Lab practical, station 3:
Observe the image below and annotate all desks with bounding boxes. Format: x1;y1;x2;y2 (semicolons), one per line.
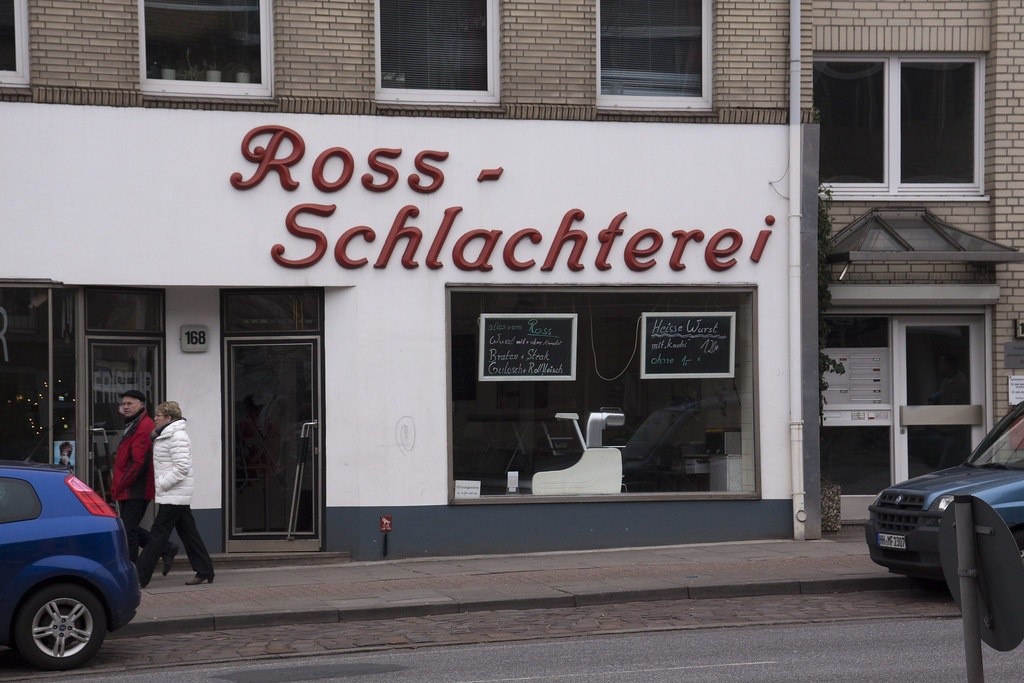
466;405;548;477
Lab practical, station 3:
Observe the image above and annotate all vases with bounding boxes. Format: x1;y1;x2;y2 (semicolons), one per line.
160;68;175;79
206;70;221;81
236;71;250;82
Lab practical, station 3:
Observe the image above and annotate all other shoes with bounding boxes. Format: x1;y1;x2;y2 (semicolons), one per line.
161;544;179;576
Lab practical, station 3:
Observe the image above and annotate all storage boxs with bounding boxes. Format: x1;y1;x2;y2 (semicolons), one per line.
704;427;741;454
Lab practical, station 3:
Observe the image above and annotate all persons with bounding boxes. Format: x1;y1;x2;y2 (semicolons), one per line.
58;442;74;470
138;400;217;589
108;388;179;576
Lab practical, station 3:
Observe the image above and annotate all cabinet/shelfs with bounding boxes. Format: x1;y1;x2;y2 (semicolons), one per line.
686;453;744;493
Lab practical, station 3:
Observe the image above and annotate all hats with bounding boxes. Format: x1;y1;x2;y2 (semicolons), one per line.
119;390;145;403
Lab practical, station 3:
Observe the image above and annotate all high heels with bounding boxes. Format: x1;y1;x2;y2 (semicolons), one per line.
185;572;214;585
139;578;149;588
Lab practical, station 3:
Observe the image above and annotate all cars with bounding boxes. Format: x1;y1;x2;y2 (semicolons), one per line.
865;400;1023;578
0;459;142;672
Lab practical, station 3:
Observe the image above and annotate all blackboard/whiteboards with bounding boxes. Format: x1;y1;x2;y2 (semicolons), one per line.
477;314;578;381
641;312;737;381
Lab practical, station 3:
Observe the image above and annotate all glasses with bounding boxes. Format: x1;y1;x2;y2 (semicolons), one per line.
156;415;165;417
